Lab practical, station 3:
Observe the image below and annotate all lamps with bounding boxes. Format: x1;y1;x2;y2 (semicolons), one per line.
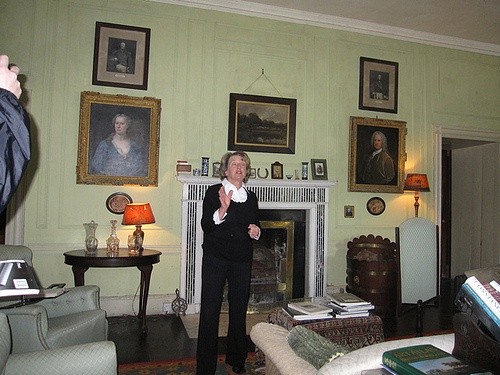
120;203;155;252
403;172;431;217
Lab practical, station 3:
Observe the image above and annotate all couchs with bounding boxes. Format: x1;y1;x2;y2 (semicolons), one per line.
249;321;455;375
0;241;118;375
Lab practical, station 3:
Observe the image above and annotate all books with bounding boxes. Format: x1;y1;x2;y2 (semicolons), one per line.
282;293;375;320
381;344;492;375
454;274;500;343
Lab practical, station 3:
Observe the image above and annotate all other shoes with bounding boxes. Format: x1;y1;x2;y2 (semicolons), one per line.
232;364;246;374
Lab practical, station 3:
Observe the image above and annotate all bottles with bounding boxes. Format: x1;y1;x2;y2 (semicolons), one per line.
83;220;98;255
107;220;120;254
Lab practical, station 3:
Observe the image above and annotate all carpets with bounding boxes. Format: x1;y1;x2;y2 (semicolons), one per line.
180;310;269;339
119;348;256;375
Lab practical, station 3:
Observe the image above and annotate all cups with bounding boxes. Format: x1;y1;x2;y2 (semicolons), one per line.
127;235;142;255
193;169;200;176
286;175;293;179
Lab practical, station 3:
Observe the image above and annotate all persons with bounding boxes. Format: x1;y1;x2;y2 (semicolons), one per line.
90;113;146;178
111;42;133;73
197;152;262;375
0;55;31;215
361;131;394;184
375;74;383;100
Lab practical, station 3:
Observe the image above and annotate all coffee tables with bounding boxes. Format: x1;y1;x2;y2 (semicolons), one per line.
265;305;385;352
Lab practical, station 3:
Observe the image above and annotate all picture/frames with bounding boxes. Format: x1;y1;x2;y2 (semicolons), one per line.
366;196;386;216
106;192;133;215
88;21;152;90
357;56;399;114
343;205;355;219
227;93;298;154
311;158;328;181
271;162;283;180
347;116;408;195
75;91;162;188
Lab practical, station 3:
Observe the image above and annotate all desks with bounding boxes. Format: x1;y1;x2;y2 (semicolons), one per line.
63;248;162;335
